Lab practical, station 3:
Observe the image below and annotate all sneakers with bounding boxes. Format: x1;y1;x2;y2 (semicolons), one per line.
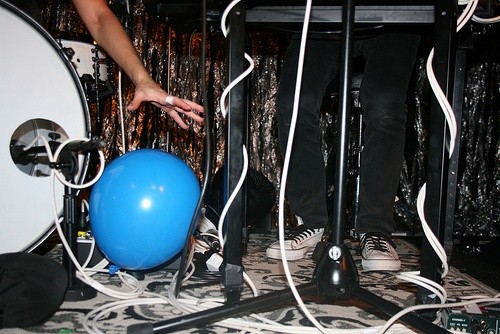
358;231;401;272
267;227;325;260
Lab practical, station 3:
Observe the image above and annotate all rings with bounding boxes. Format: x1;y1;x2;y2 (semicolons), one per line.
166;95;174;105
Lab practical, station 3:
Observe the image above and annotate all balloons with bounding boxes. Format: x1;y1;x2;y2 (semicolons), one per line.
89;149;202;276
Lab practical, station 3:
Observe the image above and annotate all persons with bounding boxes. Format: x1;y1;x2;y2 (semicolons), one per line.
73;0;204;130
265;0;425;271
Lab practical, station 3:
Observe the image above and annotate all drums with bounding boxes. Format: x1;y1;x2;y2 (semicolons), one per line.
54;32;116;102
0;0;92;255
